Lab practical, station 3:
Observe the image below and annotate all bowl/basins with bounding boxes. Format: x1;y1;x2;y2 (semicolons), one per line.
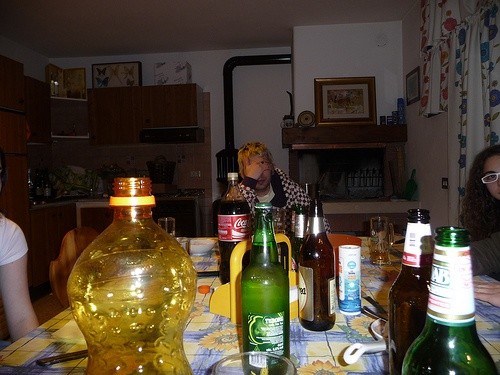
327;233;362;276
189;240;215;256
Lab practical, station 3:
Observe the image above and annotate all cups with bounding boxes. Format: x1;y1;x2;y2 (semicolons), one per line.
157;216;176;238
369;217;394;265
211;350;297;375
380;98;405;125
280;118;293;128
272;208;286;234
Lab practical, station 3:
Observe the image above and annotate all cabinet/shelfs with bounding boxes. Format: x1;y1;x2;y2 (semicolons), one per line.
0;54;212;295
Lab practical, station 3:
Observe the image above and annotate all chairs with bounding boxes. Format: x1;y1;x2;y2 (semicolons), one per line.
50;227;97;310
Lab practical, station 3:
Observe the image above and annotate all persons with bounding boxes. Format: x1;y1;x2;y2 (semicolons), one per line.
0;214;40;350
458;144;500;307
227;142;331;234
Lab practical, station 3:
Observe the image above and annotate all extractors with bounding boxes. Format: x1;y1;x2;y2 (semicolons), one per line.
138;127;205;144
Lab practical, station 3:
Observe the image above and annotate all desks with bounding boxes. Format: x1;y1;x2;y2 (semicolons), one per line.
0;233;500;374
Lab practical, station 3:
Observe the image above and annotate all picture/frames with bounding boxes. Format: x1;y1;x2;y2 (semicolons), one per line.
313;77;377;125
91;61;142;87
406;65;420;105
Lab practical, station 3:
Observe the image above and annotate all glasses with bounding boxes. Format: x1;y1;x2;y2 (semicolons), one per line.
481;173;500;184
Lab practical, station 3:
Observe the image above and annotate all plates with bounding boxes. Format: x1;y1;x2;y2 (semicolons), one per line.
298;111;315;127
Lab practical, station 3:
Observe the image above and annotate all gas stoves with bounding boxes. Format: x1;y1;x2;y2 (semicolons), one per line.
152;188;204;201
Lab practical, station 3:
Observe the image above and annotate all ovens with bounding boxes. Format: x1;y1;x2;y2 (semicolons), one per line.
152;201;200;239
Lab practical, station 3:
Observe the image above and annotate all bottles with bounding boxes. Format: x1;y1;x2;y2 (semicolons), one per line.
298;199;337;332
65;176;197;375
27;168;53;206
217;172;251;285
303;181;312;197
292;206;307;272
242;202;290;375
388;208;433;375
71;120;78;136
291;203;301;247
402;226;499;375
